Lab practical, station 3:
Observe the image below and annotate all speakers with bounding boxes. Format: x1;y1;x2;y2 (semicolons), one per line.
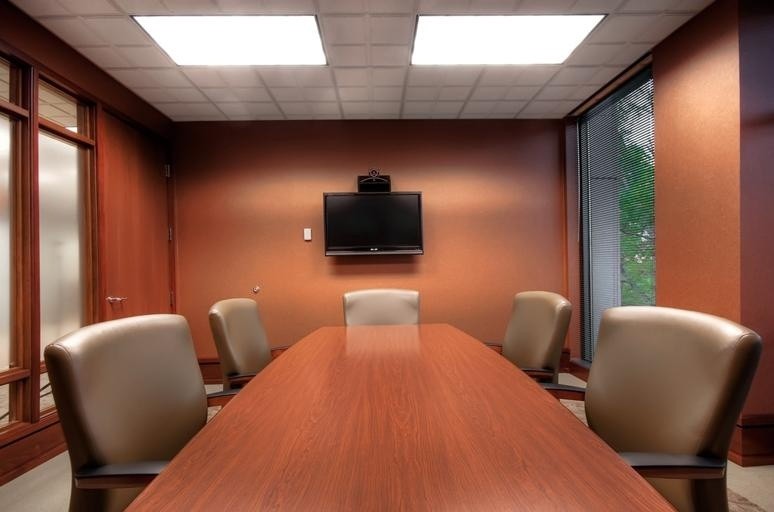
358;175;391;192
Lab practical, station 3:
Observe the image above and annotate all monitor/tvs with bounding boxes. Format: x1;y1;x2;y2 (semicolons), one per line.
323;192;424;256
